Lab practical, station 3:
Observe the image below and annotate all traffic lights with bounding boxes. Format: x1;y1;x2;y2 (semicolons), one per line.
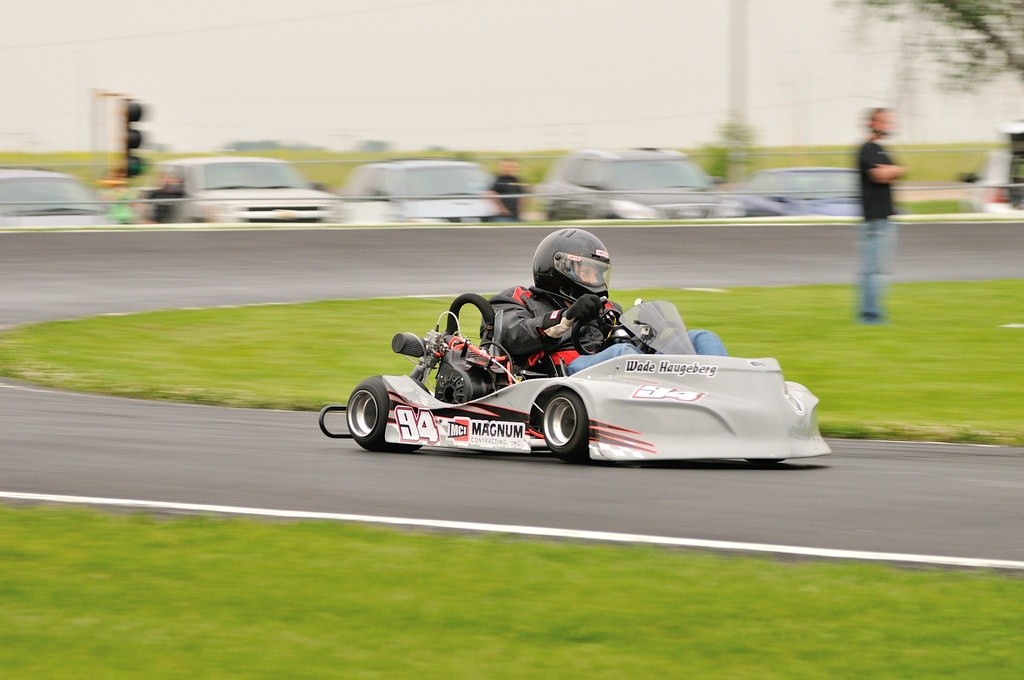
108;96;144;178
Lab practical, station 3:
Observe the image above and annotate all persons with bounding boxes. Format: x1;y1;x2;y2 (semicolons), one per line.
858;106;908;326
487;160;532;222
480;227;728;377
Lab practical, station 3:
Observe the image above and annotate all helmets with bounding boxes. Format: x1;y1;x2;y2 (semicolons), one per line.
533;229;610;305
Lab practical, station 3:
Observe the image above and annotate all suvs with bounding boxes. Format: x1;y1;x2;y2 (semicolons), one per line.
539;138;751;218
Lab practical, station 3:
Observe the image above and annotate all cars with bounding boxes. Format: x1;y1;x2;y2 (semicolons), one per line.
744;162;899;216
148;157;348;227
1;165;117;226
979;119;1024;214
341;160;511;226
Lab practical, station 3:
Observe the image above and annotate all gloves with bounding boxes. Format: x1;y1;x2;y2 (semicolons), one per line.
566;294;602;326
637;303;665;338
